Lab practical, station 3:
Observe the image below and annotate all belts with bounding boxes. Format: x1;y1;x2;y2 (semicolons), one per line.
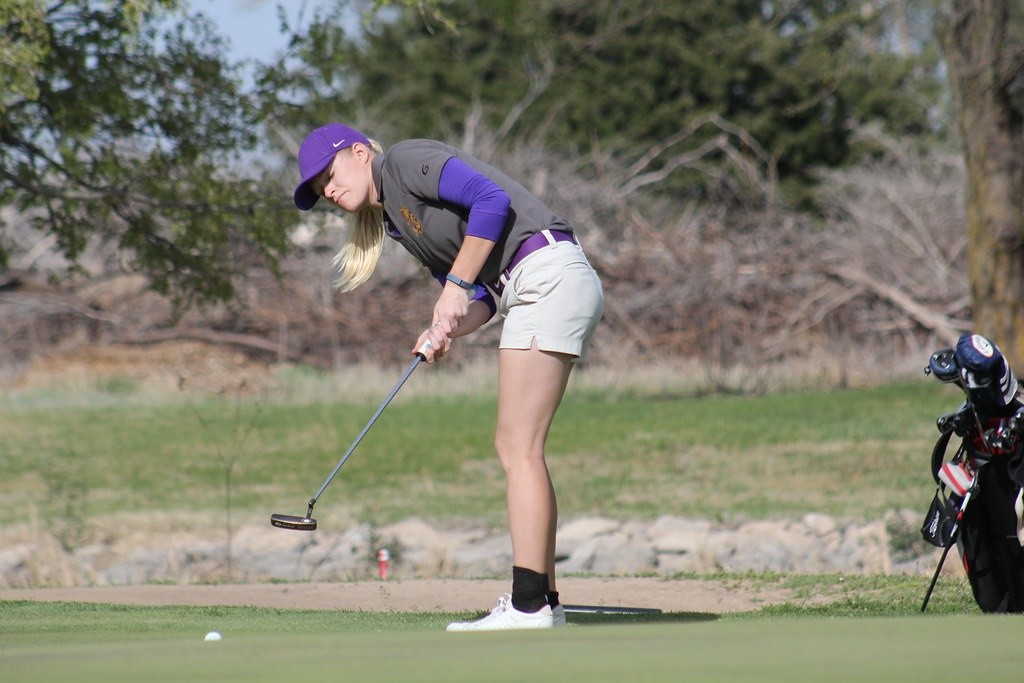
489;228;578;298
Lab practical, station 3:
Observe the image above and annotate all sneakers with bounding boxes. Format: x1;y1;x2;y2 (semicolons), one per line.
550;603;566;626
445;591;554;632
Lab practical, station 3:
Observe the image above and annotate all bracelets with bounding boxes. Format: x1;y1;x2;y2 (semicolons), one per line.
446;274;472;290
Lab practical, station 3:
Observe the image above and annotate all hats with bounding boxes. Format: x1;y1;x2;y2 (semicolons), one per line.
293;123;369;211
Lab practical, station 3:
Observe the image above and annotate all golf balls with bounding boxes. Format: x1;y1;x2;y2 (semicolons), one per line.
204;632;221;641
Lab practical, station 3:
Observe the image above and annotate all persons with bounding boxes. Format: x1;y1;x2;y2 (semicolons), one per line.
293;123;603;631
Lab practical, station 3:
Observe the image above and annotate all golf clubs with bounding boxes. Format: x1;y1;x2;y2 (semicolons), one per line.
270;338;433;532
928;331;1024;458
